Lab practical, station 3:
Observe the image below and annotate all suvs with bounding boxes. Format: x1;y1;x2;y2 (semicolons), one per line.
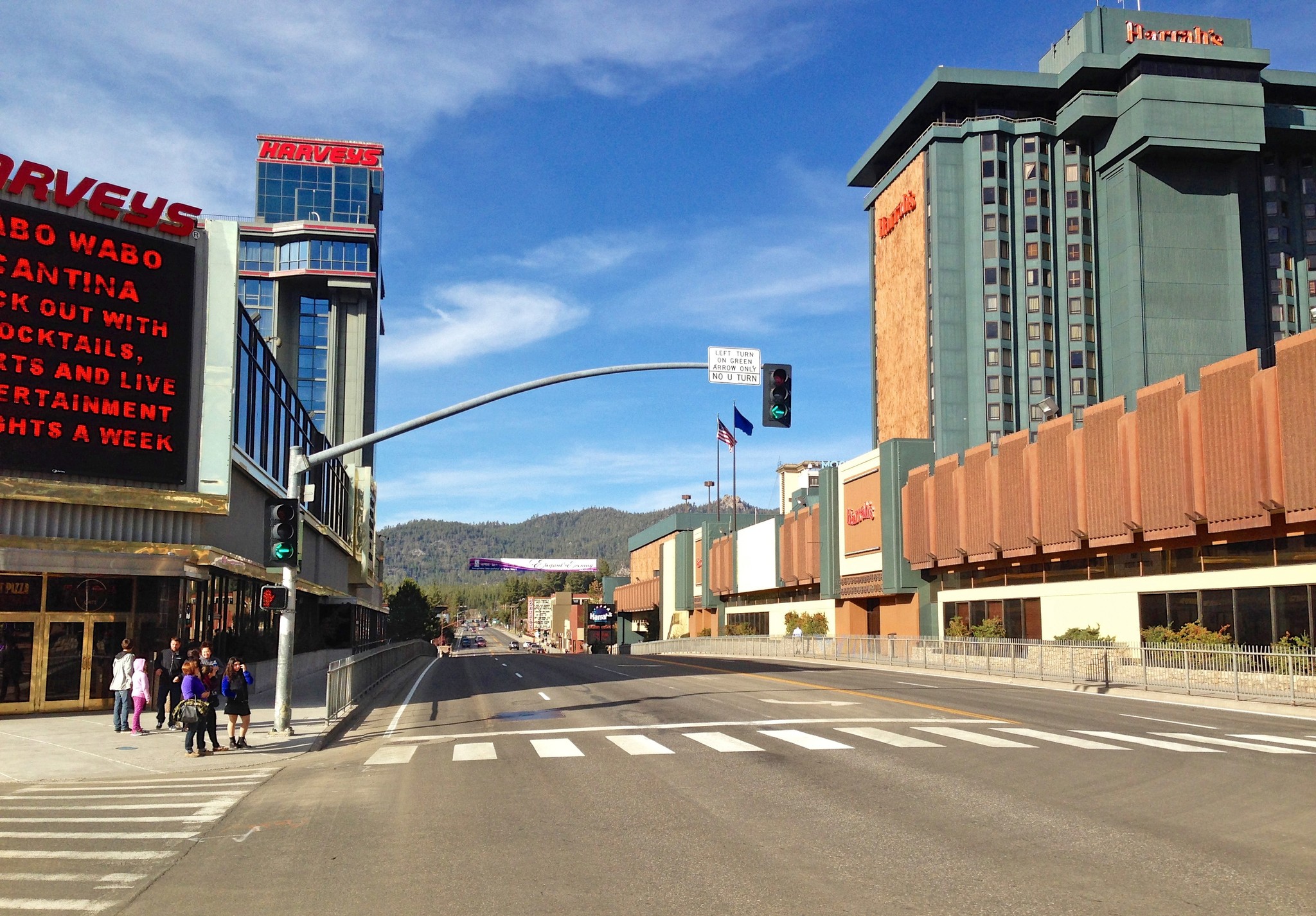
509;641;519;650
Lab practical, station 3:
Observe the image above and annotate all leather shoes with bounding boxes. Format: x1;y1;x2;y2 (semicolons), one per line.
198;750;214;756
185;751;200;758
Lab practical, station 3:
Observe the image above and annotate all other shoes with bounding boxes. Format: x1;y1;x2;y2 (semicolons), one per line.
212;746;228;752
182;727;188;732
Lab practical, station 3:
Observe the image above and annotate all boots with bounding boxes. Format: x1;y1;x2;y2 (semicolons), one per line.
230;736;240;749
237;736;252;748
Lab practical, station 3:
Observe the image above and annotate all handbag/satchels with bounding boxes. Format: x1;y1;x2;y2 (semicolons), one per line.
177;693;199;724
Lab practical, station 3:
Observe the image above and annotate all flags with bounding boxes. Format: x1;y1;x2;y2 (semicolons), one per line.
734;406;753;436
717;418;738;453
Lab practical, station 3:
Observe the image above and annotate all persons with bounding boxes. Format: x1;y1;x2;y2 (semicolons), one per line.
621;642;625;645
448;646;451;652
221;656;252;749
198;640;224;679
792;625;804;654
547;641;557;648
182;648;202;679
153;636;186;731
181;660;214;758
130;657;151;737
638;640;643;643
541;638;544;643
517;631;522;636
198;665;228;752
109;637;136;734
0;625;24;703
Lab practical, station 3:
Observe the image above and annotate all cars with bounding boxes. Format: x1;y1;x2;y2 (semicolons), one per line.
475;636;487;648
461;636;470;648
523;641;544;654
464;625;486;633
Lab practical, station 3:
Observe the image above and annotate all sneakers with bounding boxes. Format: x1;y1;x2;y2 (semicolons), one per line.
139;729;150;734
115;729;121;733
169;725;177;731
131;731;143;736
156;722;163;730
120;728;133;733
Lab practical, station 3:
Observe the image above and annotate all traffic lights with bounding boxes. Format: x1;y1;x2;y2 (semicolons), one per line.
263;497;298;568
260;585;289;610
762;362;793;427
